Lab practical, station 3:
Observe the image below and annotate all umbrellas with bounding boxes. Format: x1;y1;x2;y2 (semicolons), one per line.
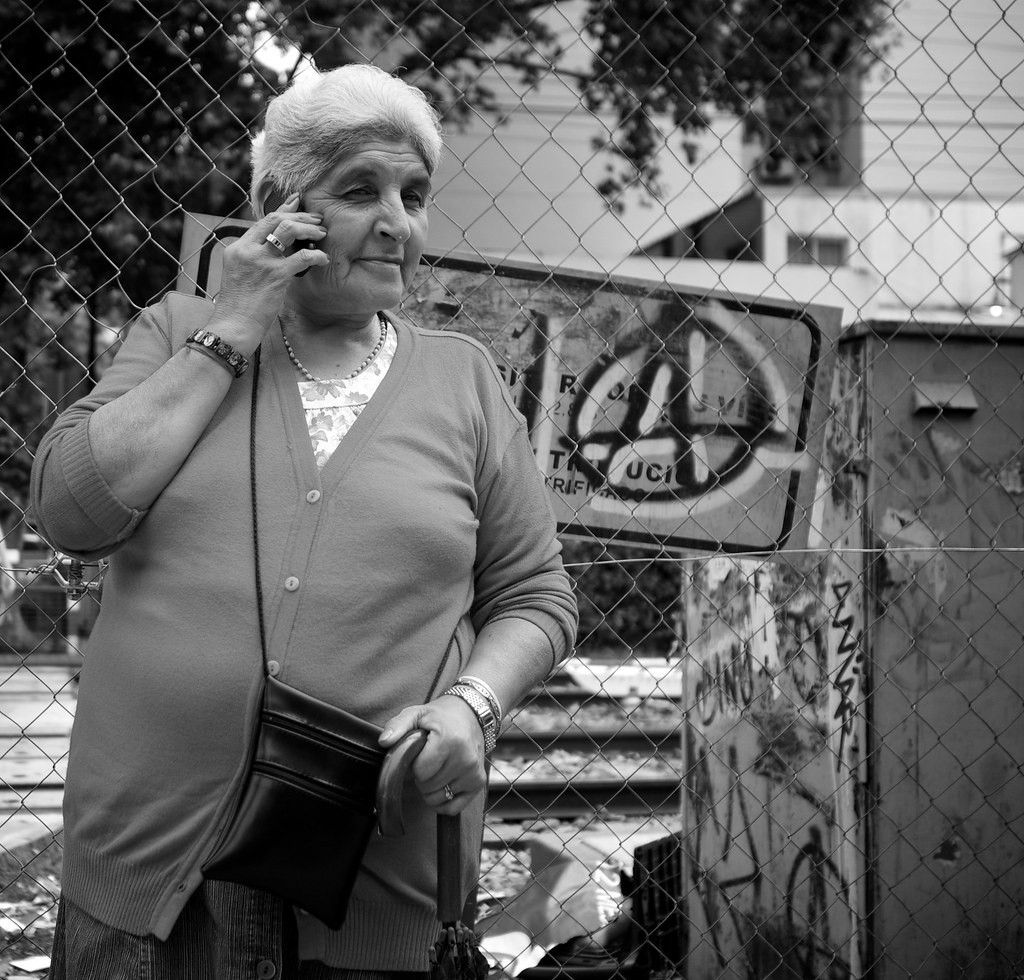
376;728;490;980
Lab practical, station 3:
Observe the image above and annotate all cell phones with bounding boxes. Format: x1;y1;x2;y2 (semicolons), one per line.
264;188;317;278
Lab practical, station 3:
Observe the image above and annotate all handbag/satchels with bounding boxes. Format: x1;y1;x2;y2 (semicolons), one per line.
199;675;398;933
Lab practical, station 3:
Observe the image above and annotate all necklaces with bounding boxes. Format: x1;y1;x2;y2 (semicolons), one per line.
283;317;385;381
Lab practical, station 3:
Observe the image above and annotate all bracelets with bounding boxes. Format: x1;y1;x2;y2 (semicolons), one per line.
182;327;250;381
444;675;502;756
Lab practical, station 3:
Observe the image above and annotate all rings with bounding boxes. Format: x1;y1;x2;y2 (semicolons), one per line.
266;233;286;253
444;784;456;800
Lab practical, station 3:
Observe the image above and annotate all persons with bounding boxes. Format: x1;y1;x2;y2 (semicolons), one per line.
29;63;579;980
